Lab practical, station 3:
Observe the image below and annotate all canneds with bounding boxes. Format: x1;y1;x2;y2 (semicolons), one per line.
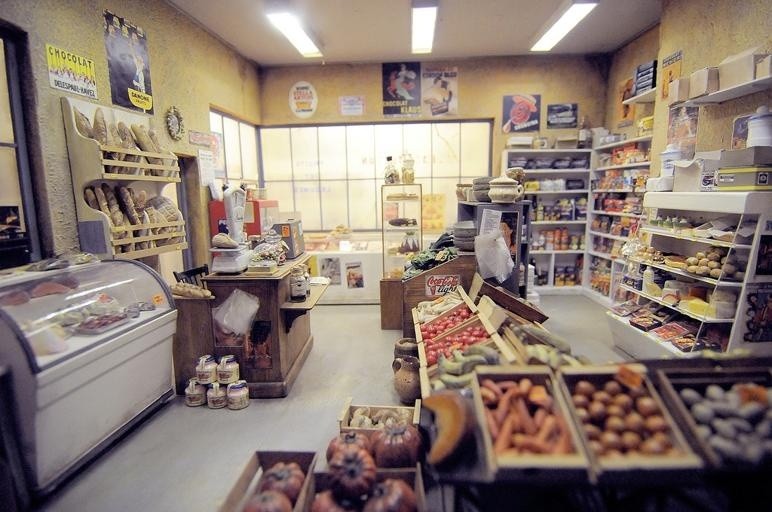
184;355;250;410
540;230;554;250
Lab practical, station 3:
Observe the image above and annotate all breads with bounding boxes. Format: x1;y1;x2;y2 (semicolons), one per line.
69;107;185;255
211;233;239;248
687;246;738;279
170;283;212;299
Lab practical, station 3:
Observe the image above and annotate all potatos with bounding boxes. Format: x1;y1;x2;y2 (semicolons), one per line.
572;381;672;460
681;383;772;462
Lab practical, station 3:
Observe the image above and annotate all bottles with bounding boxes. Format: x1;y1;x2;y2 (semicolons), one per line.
530;256;547;284
529;197;544;221
577;115;591;146
456;184;473;201
542;227;584;250
642;264;654;291
385;153;414;182
291;264;310;304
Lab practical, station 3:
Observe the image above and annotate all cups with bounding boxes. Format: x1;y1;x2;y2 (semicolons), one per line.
488;176;524;204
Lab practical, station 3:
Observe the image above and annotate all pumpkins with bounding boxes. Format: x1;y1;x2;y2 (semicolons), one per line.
244;422;423;512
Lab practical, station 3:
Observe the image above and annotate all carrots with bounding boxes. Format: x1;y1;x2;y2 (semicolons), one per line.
479;378;572;454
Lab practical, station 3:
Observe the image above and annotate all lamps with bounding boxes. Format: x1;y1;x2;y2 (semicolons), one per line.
266;5;326;59
412;3;438;54
528;1;598;52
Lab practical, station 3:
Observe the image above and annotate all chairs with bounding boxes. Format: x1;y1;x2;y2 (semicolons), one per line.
172;264;210;296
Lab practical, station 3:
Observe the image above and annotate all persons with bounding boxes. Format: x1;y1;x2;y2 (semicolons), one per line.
424;77;451;105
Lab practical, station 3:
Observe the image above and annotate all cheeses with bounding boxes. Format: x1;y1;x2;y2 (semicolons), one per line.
644;277;736;318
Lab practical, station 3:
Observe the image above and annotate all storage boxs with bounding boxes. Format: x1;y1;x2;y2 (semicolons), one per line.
667;49;771;106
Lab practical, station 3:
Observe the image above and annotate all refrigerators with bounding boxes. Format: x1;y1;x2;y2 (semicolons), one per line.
3;257;177;489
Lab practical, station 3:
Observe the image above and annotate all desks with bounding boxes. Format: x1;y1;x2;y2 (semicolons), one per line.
201;253;314;398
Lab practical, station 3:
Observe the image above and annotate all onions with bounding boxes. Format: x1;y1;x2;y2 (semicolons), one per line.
419;306;489;366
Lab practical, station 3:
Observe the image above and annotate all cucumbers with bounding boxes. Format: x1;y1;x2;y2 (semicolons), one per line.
430;345;499;392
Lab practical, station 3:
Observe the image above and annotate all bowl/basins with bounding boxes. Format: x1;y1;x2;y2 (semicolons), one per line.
512;156;587;169
472;178;492;202
452;222;477;250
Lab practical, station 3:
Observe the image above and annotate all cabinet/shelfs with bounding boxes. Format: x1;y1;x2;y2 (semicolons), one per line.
607;188;770;364
582;131;658;311
60;96;194;259
501;150;589;297
383;183;423;283
